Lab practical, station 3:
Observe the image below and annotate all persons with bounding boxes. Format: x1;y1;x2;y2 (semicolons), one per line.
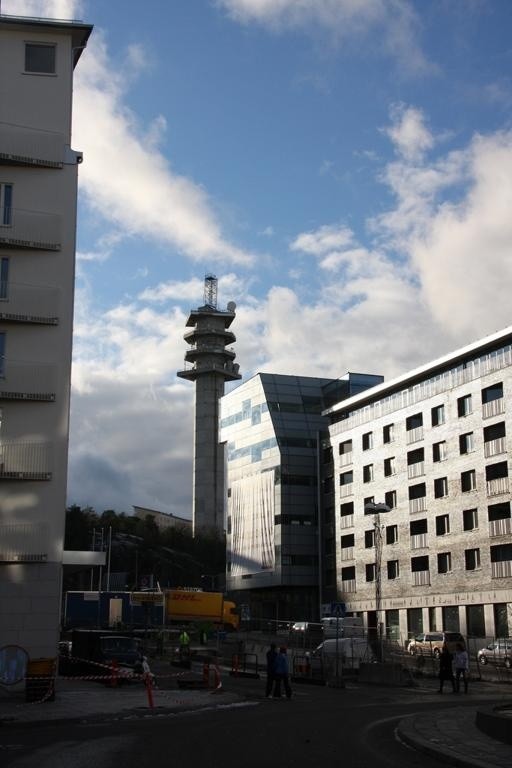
435;646;458;693
450;642;470;694
261;643;283;700
273;647;295;699
179;628;191;656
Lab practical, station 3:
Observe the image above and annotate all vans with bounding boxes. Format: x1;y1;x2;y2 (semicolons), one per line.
291;615;375;660
405;631;467;660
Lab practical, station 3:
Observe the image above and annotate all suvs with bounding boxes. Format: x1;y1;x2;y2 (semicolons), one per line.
476;636;511;667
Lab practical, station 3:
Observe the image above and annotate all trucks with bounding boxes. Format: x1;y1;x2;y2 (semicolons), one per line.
166;589;239;634
96;634;145;682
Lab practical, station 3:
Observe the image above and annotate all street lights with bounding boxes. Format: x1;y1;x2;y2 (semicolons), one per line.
364;501;391;647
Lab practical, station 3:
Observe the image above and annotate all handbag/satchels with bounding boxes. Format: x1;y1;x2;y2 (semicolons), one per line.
464;672;470;678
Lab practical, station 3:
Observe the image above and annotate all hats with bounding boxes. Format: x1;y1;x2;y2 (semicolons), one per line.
280;646;287;652
